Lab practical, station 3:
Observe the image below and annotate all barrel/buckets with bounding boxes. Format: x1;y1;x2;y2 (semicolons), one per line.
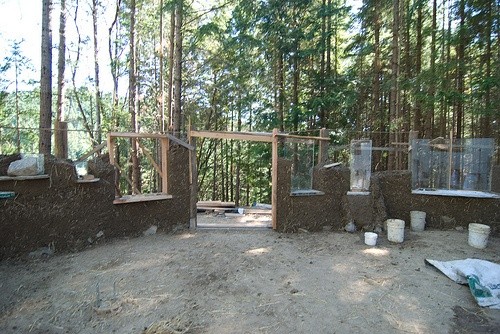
468;223;490;249
387;219;406;243
410;211;427;232
364;232;378;246
